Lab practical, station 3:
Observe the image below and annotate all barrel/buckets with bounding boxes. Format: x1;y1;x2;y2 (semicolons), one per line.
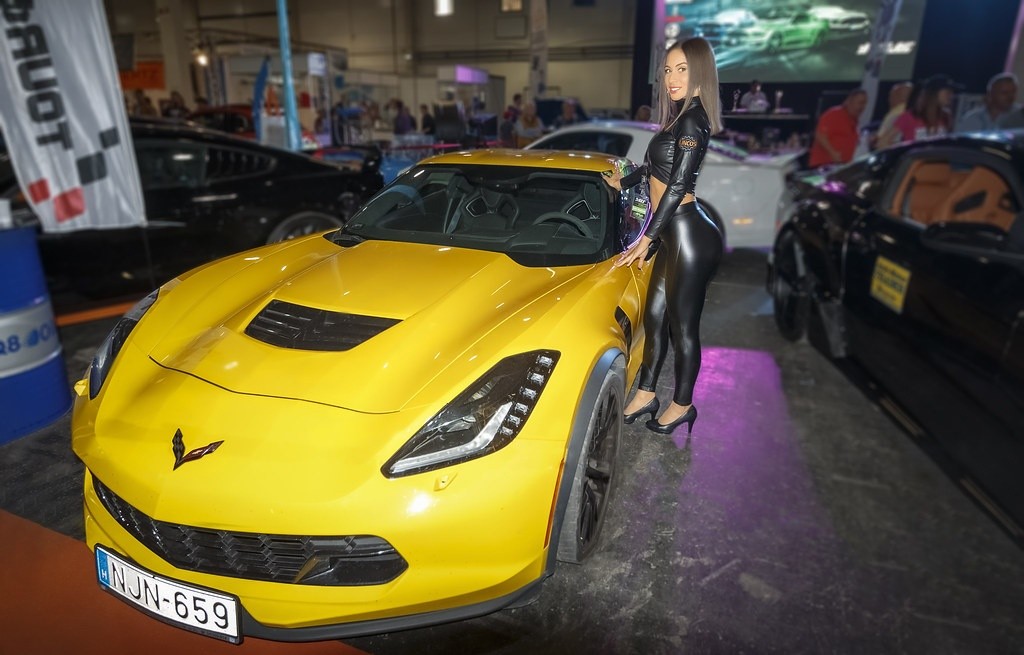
0;222;73;444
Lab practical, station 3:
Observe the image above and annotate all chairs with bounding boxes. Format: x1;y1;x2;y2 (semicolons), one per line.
560;182;600;238
904;165;1008;228
453;187;520;235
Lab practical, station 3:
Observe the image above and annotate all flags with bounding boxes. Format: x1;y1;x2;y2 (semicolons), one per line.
0;0;148;233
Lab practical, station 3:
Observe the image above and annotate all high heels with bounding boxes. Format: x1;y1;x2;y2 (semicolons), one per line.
621;396;660;424
646;405;697;434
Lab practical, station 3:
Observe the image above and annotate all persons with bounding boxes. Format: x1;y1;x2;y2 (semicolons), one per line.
124;86;582;150
742;72;1024;168
602;37;724;435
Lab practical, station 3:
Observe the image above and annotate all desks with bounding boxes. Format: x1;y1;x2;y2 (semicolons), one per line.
385;144;461;155
477;141;509;148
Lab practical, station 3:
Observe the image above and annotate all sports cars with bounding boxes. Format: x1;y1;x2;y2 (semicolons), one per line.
72;146;661;646
523;120;808;254
767;129;1024;553
0;117;382;315
